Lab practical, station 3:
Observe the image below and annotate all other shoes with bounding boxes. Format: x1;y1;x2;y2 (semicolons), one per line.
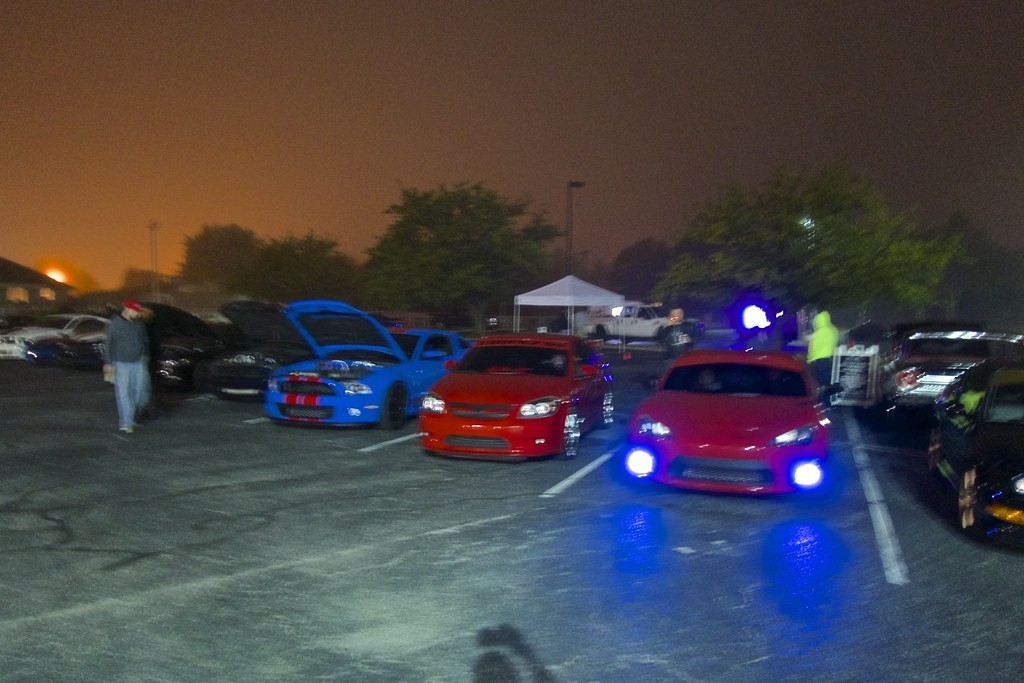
822;406;831;413
120;427;134;434
133;422;145;429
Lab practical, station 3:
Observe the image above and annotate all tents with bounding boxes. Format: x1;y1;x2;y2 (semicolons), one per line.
513;274;626;356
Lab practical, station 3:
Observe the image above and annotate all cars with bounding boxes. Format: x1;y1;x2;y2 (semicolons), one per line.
417;332;613;459
215;298;316;404
0;313;111;361
630;347;843;495
24;304;208;372
150;332;233;387
265;299;478;429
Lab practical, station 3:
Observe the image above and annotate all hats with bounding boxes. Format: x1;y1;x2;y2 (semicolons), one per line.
125;301;144;312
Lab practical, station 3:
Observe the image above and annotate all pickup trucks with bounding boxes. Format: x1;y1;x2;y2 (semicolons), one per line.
574;304;702;347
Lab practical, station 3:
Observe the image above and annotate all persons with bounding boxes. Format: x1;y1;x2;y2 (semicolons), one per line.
806;310;839;413
657;305;697;363
105;301;150;433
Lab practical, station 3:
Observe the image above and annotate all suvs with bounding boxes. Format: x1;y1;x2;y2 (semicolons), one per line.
877;322;991;430
928;359;1024;540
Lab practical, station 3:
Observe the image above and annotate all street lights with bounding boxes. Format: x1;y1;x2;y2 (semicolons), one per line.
564;181;586;274
